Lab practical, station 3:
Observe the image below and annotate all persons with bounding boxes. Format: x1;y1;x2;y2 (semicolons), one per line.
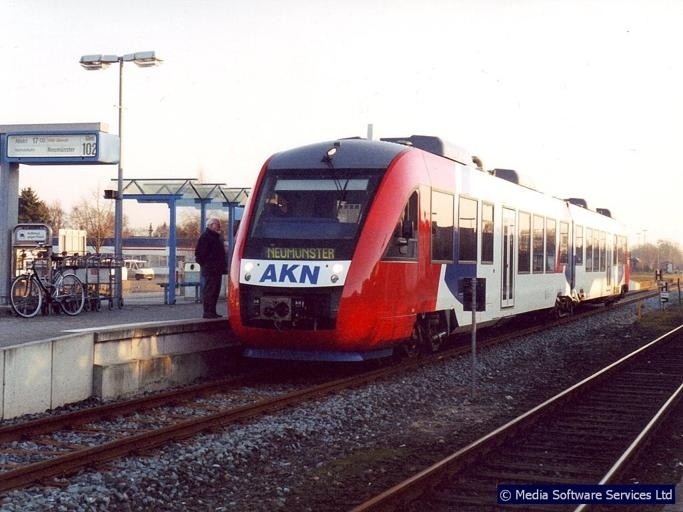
194;218;225;319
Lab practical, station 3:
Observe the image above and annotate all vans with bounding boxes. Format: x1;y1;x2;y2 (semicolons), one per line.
122;260;155;281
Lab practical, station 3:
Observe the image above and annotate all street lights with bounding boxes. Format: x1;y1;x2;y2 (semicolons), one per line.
78;50;165;305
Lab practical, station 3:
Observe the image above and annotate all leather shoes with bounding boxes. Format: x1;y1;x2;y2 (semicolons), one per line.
203;313;223;319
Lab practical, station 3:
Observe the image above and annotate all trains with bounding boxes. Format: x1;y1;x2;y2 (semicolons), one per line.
226;135;631;382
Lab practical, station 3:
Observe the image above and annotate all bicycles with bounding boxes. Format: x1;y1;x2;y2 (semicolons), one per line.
10;258;85;318
